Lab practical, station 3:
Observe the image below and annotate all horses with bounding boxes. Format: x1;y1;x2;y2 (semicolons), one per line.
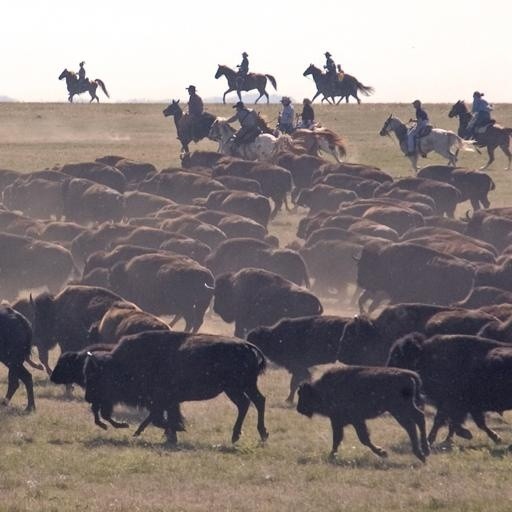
302;64;373;104
447;99;512;170
58;68;109;104
378;113;477;172
215;64;277;105
206;111;346;159
162;98;215;153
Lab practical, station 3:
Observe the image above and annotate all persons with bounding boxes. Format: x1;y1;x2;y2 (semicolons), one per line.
235;52;249;89
405;100;430;156
338;64;344;74
224;101;256;153
76;62;85;86
324;52;337;78
297;99;315;128
277;97;294;133
464;92;492;144
184;86;203;121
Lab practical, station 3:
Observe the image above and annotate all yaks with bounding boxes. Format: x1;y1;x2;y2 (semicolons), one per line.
0;150;512;466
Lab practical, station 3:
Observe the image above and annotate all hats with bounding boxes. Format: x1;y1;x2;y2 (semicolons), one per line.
232;101;246;108
303;98;312;105
323;51;332;56
279;96;292;103
413;99;420;106
186;85;198;91
242;51;249;57
